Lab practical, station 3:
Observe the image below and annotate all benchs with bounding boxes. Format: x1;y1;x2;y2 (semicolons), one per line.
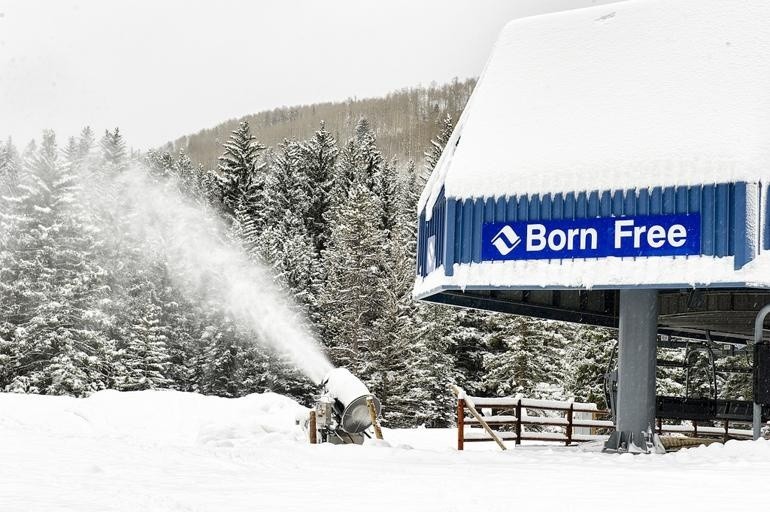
655;395;717;437
715;396;770;437
